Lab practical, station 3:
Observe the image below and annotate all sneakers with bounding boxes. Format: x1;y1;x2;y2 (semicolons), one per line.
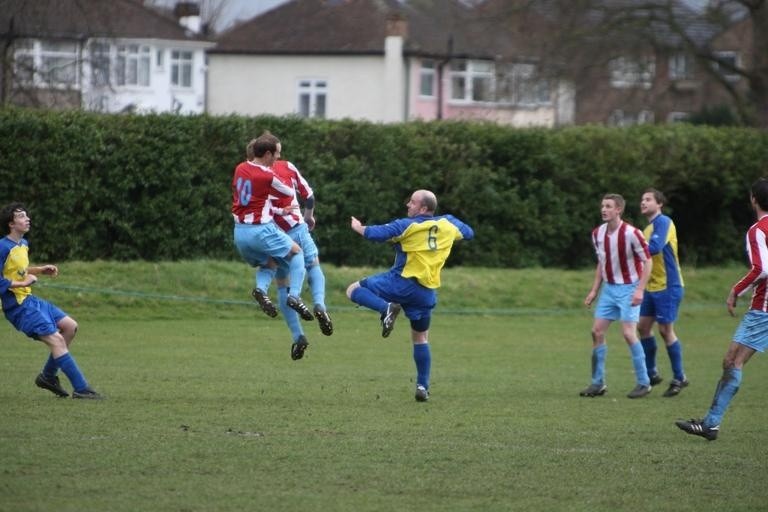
33;370;102;401
413;385;431;403
626;374;690;399
578;383;609;398
379;299;401;339
251;287;334;361
673;416;720;441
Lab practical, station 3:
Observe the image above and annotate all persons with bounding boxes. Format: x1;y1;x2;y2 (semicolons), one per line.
636;188;689;397
675;178;768;442
0;203;102;400
345;188;475;402
232;133;315;322
579;191;653;398
245;138;334;361
